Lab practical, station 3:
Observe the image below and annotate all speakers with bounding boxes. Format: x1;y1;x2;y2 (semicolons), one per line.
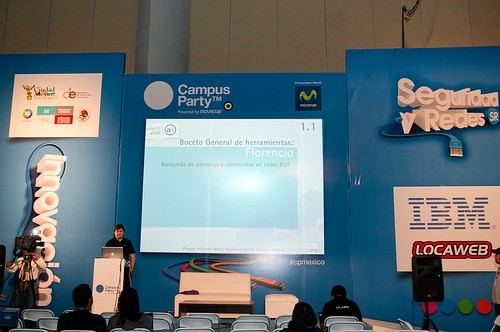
412;255;444;302
0;245;6;292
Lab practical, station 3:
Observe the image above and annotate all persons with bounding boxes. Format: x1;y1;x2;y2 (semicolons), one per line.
57;283;107;332
490;247;500;332
6;251;46;311
320;285;363;329
279;302;323;332
107;287;154;330
105;223;137;288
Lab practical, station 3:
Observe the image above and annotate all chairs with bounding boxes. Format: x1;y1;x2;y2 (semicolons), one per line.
491;315;500;332
9;309;430;332
266;294;298;318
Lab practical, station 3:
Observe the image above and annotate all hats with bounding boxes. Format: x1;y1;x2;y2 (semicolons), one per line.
491;247;500;254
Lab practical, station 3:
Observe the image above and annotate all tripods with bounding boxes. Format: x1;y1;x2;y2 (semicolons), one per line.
8;256;36;308
421;302;439;332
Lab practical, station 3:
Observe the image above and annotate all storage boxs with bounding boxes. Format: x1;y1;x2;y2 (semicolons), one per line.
2;308;20;326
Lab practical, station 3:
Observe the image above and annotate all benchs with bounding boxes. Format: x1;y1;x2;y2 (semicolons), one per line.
174;272;254;317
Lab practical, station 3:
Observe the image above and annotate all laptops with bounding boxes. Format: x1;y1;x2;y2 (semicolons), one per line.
102;247;123;259
145;312;153;331
